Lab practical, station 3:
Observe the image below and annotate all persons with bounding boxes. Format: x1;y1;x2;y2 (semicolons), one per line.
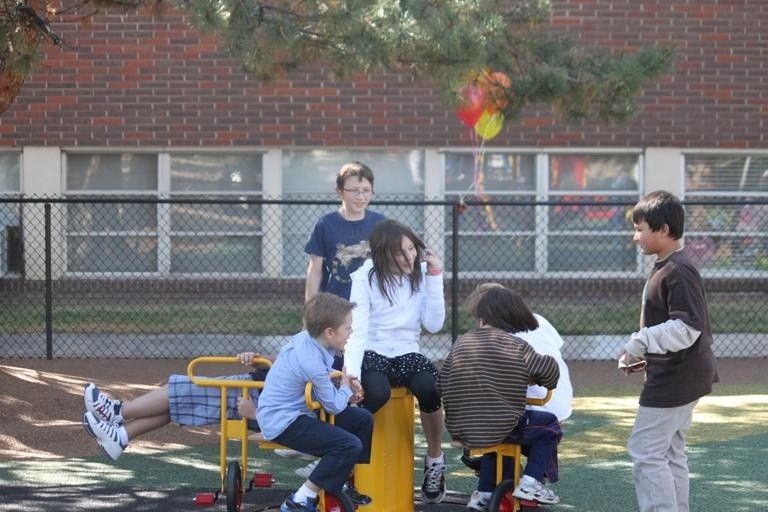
617;190;720;512
461;312;573;477
341;219;447;505
83;352;343;462
434;282;563;512
304;160;390;305
256;292;374;512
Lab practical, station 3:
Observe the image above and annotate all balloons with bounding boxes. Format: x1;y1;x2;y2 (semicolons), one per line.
453;82;486;129
479;72;513;116
474;109;504;141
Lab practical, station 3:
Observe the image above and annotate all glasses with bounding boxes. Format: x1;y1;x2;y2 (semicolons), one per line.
342;187;372;197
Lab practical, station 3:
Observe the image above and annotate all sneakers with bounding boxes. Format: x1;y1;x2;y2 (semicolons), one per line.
280;492;321;512
82;411;127;461
512;475;560;505
295;461;320;478
341;481;372;506
273;448;318;459
467;490;492;511
422;452;446;505
84;383;123;426
